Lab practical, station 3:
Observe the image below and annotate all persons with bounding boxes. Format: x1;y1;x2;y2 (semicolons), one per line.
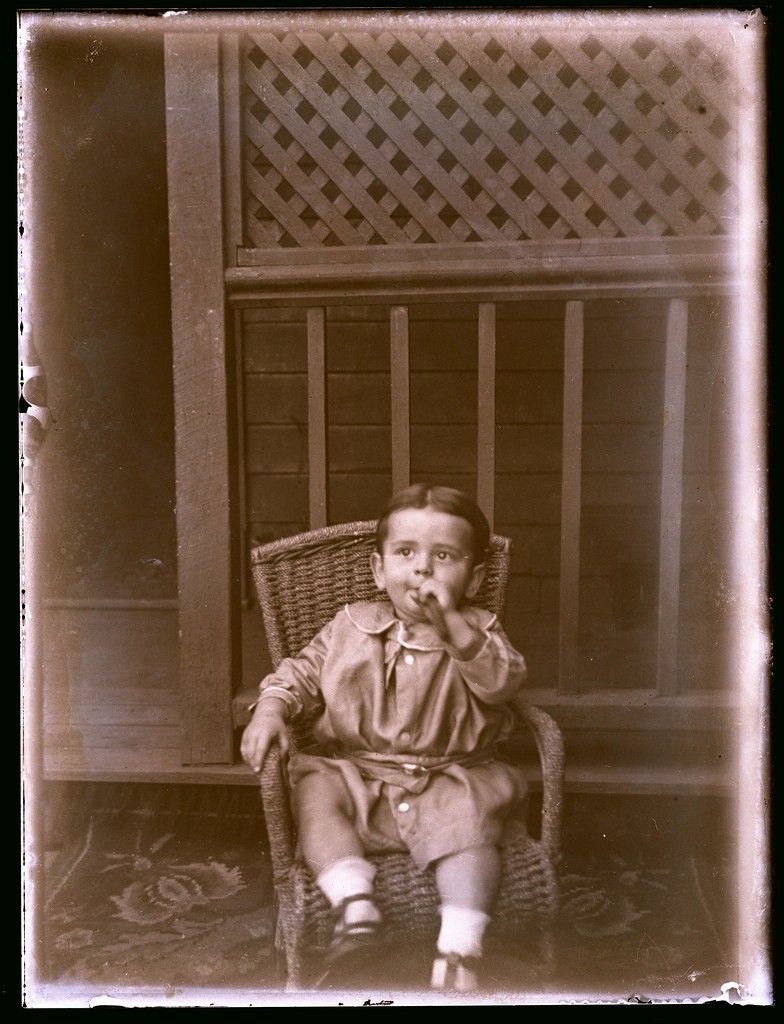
240;482;526;993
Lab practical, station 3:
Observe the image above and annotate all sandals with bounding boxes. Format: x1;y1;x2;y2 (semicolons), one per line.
319;894;392;976
430;945;483;994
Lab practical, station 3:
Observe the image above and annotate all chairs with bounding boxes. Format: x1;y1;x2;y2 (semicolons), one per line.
251;518;564;993
